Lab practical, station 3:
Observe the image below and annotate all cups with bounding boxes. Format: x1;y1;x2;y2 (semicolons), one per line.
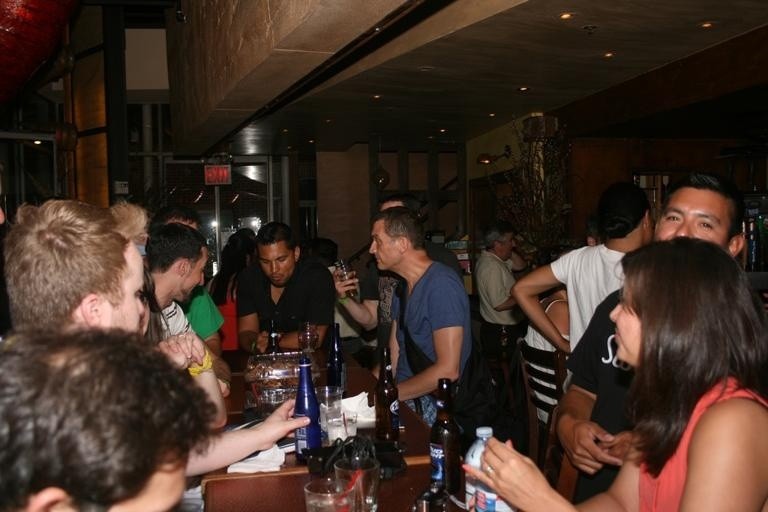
305;457;382;512
332;258;358;297
242;350;360;452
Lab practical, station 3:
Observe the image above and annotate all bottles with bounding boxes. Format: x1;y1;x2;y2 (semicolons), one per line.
324;321;347;397
428;377;460;495
295;356;321;462
295;321;319;356
265;319;283;354
464;424;521;512
414;481;450;511
372;347;403;468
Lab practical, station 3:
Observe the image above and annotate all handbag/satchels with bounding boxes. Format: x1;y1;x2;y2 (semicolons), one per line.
482;338;527;454
397;277;495;418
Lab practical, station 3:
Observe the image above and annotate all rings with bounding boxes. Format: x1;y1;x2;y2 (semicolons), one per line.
485;466;494;475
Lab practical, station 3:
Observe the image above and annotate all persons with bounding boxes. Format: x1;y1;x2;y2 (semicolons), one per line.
584;213;602;247
552;167;768;504
516;245;577;426
475;226;525;420
0;198;312;479
0;327;218;512
140;204;337;397
510;179;655;355
461;234;768;511
332;193;473;417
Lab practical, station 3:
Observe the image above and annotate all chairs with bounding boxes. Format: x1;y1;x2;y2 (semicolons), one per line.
480;325;580;505
